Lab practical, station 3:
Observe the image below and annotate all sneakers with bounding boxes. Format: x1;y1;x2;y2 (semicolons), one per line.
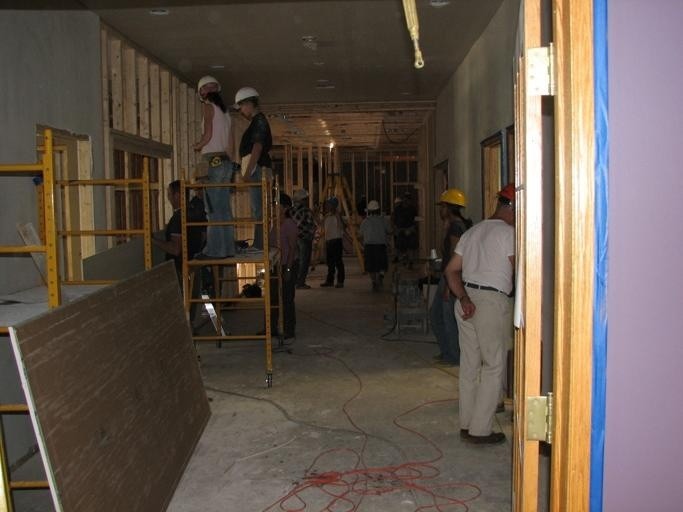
297;284;311;289
460;429;505;444
320;283;343;288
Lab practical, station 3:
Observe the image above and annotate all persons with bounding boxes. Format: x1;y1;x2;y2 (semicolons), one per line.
150;179;315;350
358;195;416;294
231;86;279;256
496;267;516;424
439;182;520;450
427;188;475;366
190;74;238;262
316;198;346;287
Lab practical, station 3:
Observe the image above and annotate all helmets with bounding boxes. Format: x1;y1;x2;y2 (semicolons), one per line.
198;76;259;103
328;197;338;207
498;184;515;202
367;201;379;210
294;189;307;201
440;189;465;207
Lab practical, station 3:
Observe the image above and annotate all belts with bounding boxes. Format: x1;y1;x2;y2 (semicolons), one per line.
463;280;499;291
